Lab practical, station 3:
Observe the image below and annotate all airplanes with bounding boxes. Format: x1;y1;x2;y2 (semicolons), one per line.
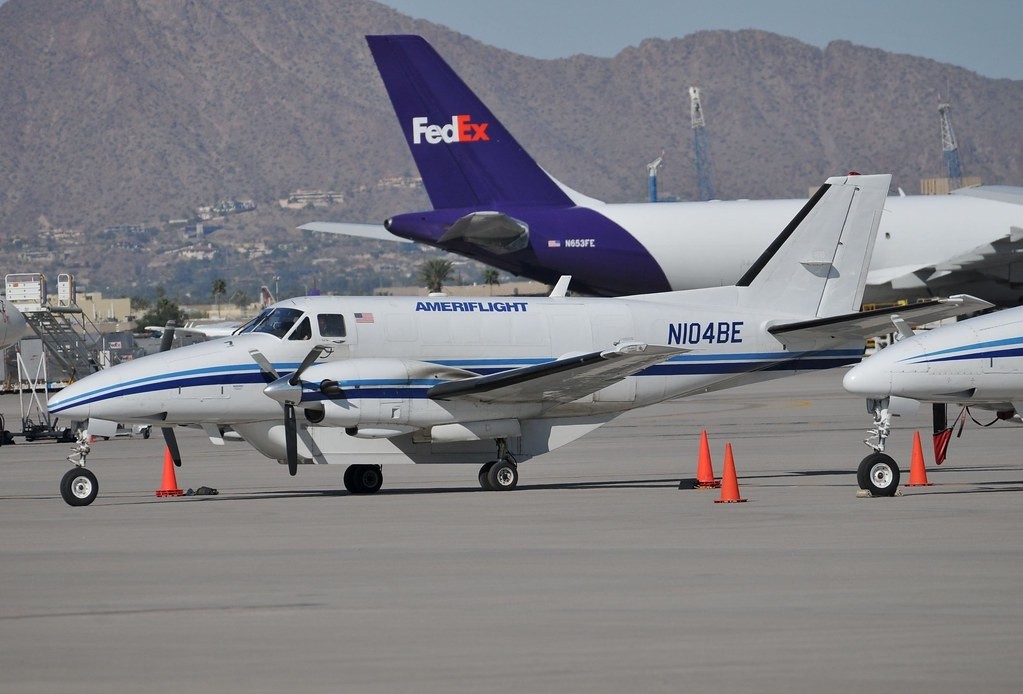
843;304;1023;497
43;172;994;508
299;33;1023;297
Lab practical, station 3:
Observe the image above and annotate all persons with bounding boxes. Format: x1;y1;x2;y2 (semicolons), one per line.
319;318;330;335
289;316;309;340
262;295;270;310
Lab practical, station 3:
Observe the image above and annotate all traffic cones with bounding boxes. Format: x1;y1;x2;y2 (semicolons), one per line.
156;444;183;497
905;430;934;487
714;442;748;503
695;429;722;488
